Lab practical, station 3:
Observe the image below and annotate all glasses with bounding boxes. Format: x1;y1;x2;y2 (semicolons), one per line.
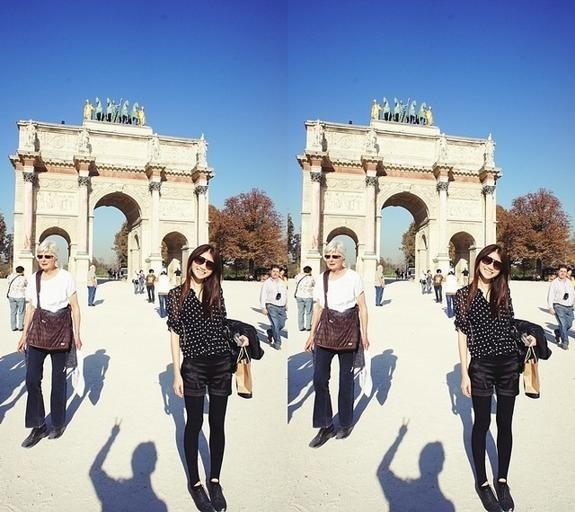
482;255;503;270
324;254;341;259
37;255;53;259
194;255;216;271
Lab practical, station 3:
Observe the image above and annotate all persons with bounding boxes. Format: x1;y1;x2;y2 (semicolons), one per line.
279;268;288;288
262;265;289;350
453;245;537;512
371;97;433;126
566;268;575;287
107;268;124;281
395;268;411;280
84;98;145;126
7;266;27;331
420;268;459;317
17;241;82;448
86;265;97;306
375;263;385;306
547;265;575;350
165;245;250;512
134;269;169;317
293;267;315;331
303;241;369;448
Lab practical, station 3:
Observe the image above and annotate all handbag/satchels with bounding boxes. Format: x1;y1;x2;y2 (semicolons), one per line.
26;306;72;351
523;345;540;395
314;303;359;351
235;347;253;394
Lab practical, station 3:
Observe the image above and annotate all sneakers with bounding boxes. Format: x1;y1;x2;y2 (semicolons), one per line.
190;483;214;511
21;423;49;447
206;481;226;512
267;329;273;342
335;423;353;438
48;424;65;439
477;482;502;511
309;424;335;448
554;329;561;341
495;478;514;511
